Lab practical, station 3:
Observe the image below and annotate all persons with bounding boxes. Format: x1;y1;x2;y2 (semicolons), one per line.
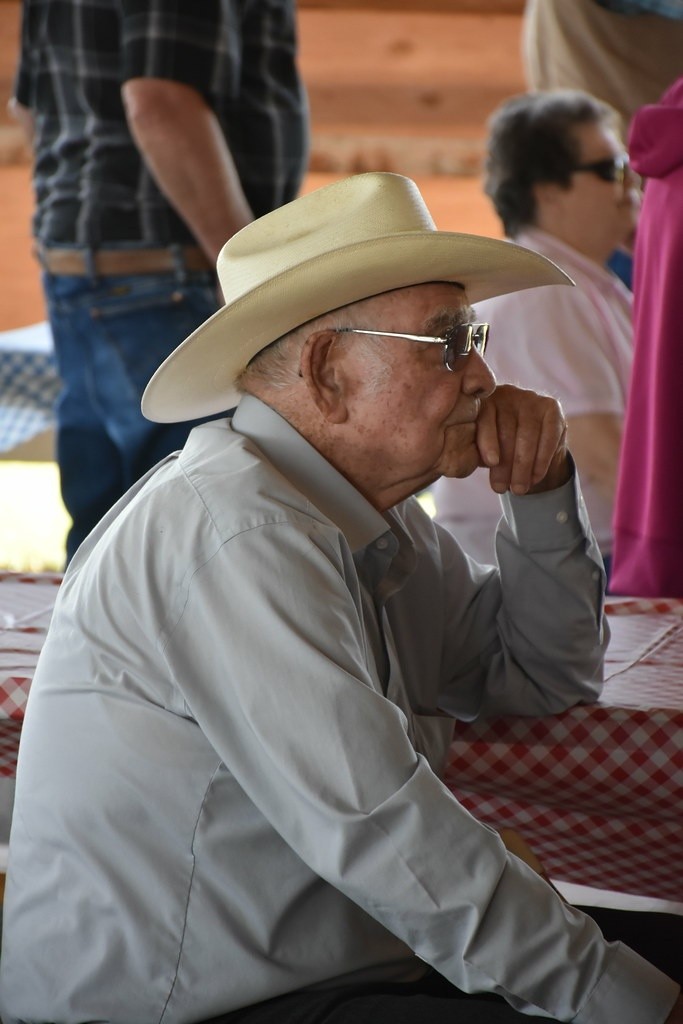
8;0;308;572
608;78;683;602
0;171;683;1024
524;0;683;291
434;91;645;597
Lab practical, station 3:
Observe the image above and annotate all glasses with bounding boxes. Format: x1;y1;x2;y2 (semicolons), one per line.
573;154;628;183
299;323;490;377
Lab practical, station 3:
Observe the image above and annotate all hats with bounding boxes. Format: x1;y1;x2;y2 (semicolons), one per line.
141;172;575;424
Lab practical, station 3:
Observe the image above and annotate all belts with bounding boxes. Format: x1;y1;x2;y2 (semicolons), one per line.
43;246;210;276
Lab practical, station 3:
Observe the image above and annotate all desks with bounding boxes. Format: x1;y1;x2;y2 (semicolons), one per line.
0;575;682;902
